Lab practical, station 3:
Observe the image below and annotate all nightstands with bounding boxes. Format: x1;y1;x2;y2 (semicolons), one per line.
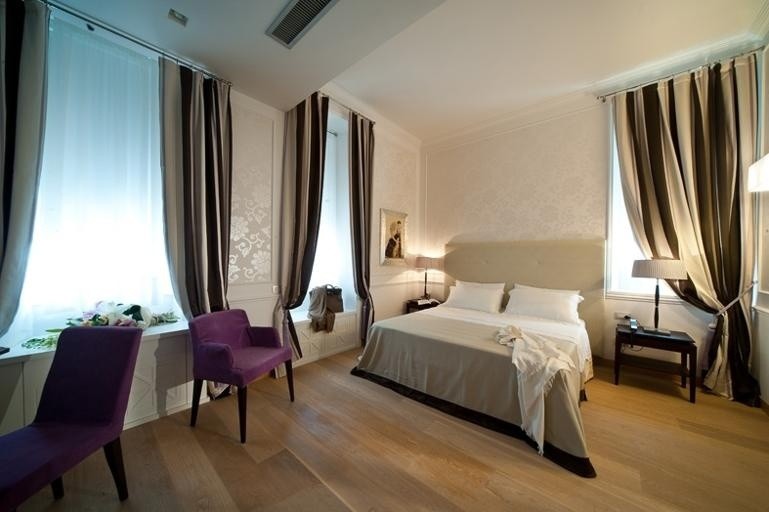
371;239;607;478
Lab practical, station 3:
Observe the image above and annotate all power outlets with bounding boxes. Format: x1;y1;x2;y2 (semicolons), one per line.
615;312;635;319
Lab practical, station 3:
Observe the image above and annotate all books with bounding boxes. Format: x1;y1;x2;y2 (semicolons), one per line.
416;298;440;306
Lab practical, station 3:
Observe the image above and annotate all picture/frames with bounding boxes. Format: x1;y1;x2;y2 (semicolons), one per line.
379;208;409;266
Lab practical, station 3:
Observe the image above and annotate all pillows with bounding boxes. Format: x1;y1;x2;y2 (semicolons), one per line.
440;280;584;326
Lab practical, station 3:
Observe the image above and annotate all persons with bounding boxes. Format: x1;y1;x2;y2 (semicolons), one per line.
385;220;402;259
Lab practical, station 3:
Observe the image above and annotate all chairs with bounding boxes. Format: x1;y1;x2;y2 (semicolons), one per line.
188;309;294;443
0;327;143;512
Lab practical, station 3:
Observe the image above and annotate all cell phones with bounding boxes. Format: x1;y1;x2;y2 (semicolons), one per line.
630;318;638;331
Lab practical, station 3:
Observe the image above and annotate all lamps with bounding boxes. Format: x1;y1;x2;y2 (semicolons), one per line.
747;153;769;192
632;259;688;336
414;257;440;300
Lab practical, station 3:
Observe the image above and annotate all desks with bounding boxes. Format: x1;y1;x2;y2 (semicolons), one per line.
614;323;697;403
406;300;443;314
